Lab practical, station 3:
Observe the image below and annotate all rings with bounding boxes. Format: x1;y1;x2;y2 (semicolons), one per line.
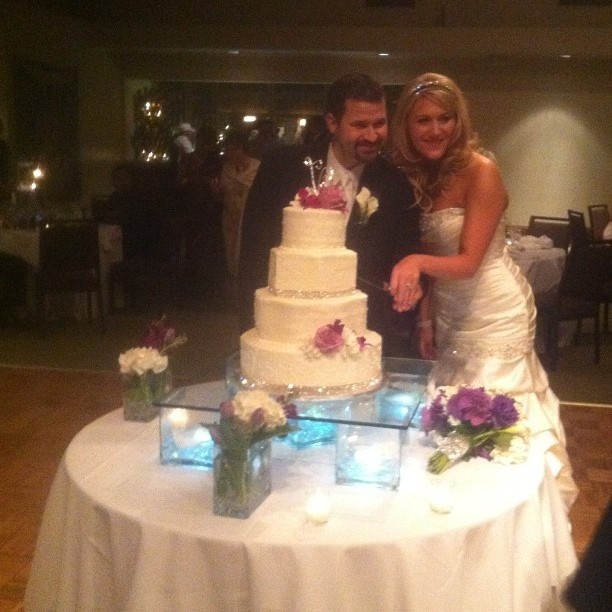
404;282;411;290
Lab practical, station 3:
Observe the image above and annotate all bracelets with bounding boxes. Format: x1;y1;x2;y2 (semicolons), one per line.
411;315;434;333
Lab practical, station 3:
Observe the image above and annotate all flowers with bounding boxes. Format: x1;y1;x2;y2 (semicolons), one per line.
117;309;189;413
293;183;355;214
199;389;302;501
420;382;532;476
300;319;383;364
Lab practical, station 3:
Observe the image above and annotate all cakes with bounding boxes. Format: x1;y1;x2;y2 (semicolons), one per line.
239;156;382;400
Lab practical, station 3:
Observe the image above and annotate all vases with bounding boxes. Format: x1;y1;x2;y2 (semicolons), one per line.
119;366;172;425
212;436;276;519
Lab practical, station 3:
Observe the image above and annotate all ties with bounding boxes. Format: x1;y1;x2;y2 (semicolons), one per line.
338;171;353;190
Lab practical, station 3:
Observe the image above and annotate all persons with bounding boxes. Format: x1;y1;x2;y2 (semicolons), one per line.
172;121;196;155
391;71;573;536
288;72;424;359
208;142;258;284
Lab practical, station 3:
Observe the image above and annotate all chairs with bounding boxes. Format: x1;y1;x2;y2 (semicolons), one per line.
503;223;529;239
564;206;590;242
585;203;612;242
30;218;110;339
528;214;602;371
562;239;611;342
